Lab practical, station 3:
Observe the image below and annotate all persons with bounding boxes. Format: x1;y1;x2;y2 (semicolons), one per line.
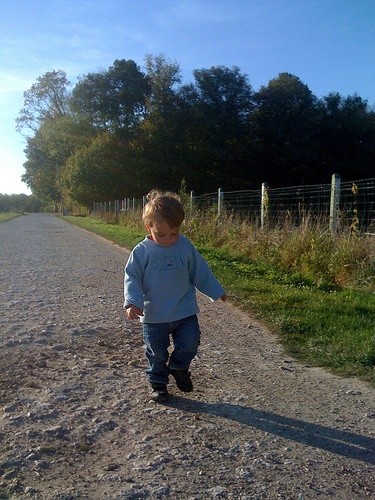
122;189;228;401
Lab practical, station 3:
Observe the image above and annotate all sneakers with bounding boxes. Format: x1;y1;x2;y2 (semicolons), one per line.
168;363;193;393
148;377;168;403
